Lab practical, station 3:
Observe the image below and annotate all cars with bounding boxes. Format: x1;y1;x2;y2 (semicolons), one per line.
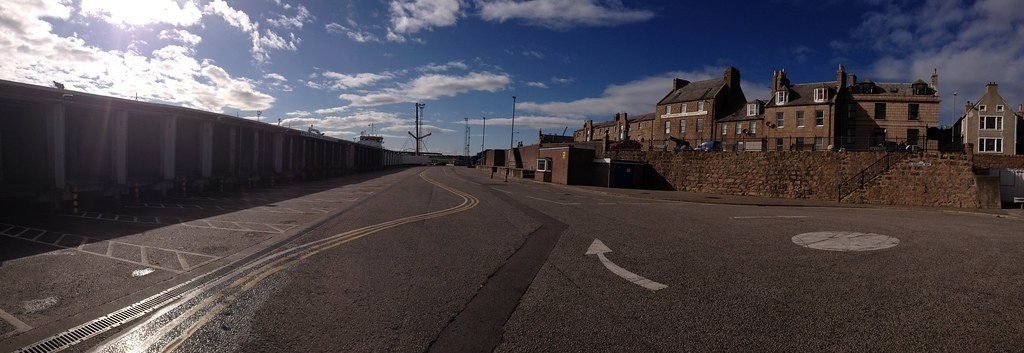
609;140;641;149
869;141;923;151
695;141;722;151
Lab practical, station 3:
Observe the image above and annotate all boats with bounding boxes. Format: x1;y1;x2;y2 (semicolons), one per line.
358;123;385;149
